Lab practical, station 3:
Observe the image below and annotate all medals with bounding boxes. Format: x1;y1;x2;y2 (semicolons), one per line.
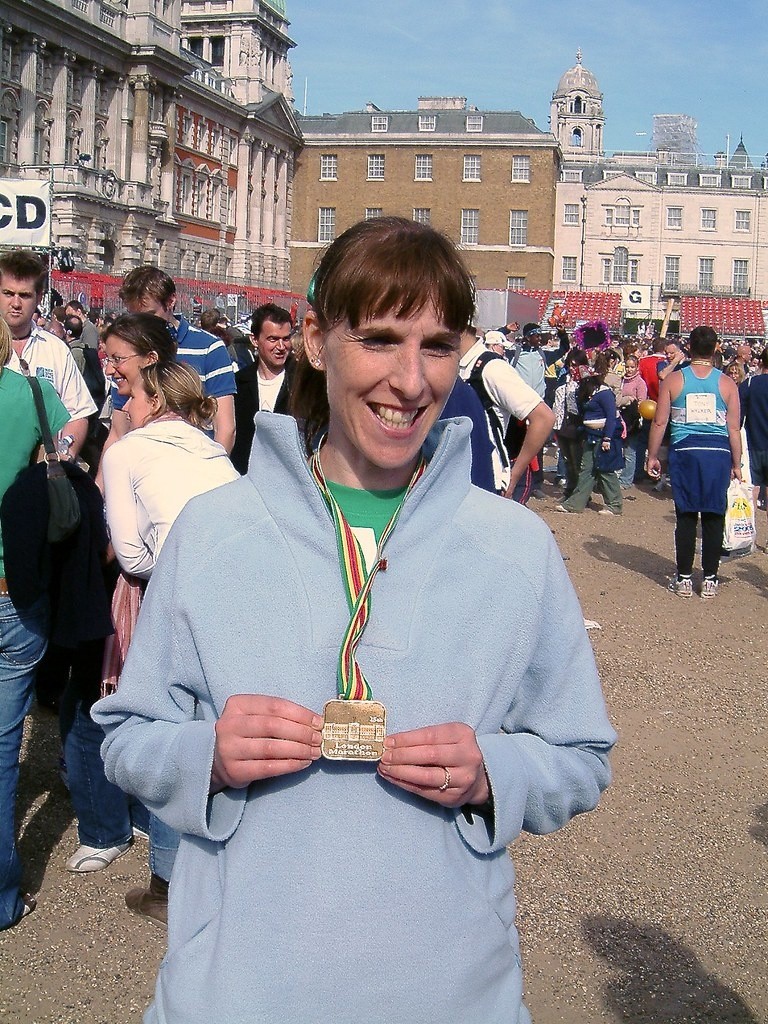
319;700;386;762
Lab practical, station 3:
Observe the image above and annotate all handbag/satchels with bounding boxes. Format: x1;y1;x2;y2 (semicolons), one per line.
0;377;107;636
558;412;584;439
721;478;757;551
594;436;624;473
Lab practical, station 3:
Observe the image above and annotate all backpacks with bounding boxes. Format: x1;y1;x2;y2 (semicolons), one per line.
467;349;528;468
71;344;105;395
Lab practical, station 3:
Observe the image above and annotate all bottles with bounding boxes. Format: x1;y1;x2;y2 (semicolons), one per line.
56;434;76;456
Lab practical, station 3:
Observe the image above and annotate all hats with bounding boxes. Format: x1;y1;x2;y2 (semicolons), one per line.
484;331;512;349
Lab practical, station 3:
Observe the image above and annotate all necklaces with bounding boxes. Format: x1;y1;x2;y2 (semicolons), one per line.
692;360;712;366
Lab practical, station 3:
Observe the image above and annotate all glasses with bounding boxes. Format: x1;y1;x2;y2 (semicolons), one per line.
100;355;140;368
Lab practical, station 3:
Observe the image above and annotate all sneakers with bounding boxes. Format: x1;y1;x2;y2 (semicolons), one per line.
66;841;134;873
667;573;692;597
700;580;719;599
124;886;170;928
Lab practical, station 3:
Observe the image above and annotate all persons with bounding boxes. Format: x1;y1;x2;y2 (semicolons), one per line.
90;215;617;1024
0;249;768;933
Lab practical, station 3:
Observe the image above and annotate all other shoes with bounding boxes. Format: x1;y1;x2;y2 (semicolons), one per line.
556;505;568;513
533;490;546;499
599;509;619;517
23;893;37;916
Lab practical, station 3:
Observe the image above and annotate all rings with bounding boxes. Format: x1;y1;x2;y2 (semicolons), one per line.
440;766;451;791
